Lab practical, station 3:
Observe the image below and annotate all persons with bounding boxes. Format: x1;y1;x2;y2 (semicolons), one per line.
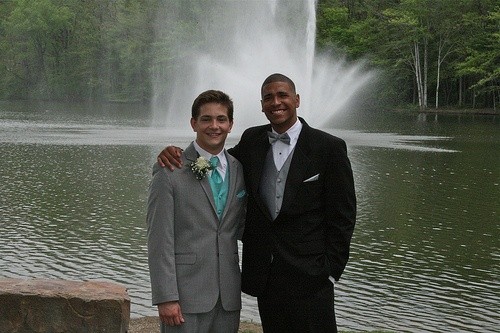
157;72;357;333
145;87;249;332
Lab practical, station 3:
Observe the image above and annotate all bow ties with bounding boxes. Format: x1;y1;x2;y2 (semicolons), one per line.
266;130;291;146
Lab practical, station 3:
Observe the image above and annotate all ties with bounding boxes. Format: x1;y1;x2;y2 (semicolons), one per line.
210;157;223;194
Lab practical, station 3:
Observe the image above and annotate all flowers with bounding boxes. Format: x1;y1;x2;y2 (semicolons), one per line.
190;155;213;180
